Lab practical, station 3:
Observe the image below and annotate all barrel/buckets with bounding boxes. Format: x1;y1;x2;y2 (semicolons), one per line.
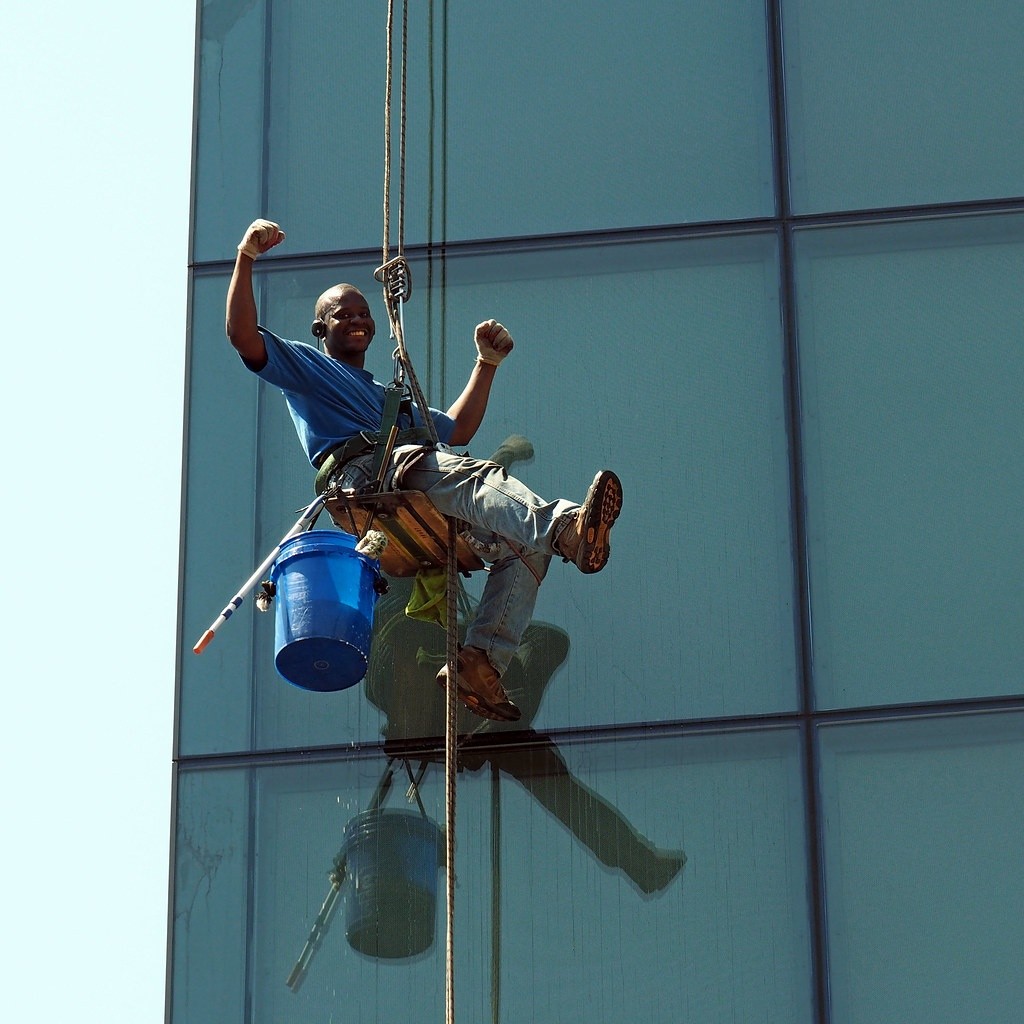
269;530;387;692
341;807;445;959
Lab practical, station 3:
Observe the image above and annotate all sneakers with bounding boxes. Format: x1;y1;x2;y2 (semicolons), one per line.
560;469;623;575
436;647;522;722
626;846;687;894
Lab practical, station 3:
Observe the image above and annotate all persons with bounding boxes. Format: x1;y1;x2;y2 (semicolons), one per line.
224;219;624;721
364;435;689;899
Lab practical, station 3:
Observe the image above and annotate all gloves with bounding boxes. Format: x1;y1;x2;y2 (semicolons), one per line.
489;435;534;470
237;219;286;261
474;319;513;365
